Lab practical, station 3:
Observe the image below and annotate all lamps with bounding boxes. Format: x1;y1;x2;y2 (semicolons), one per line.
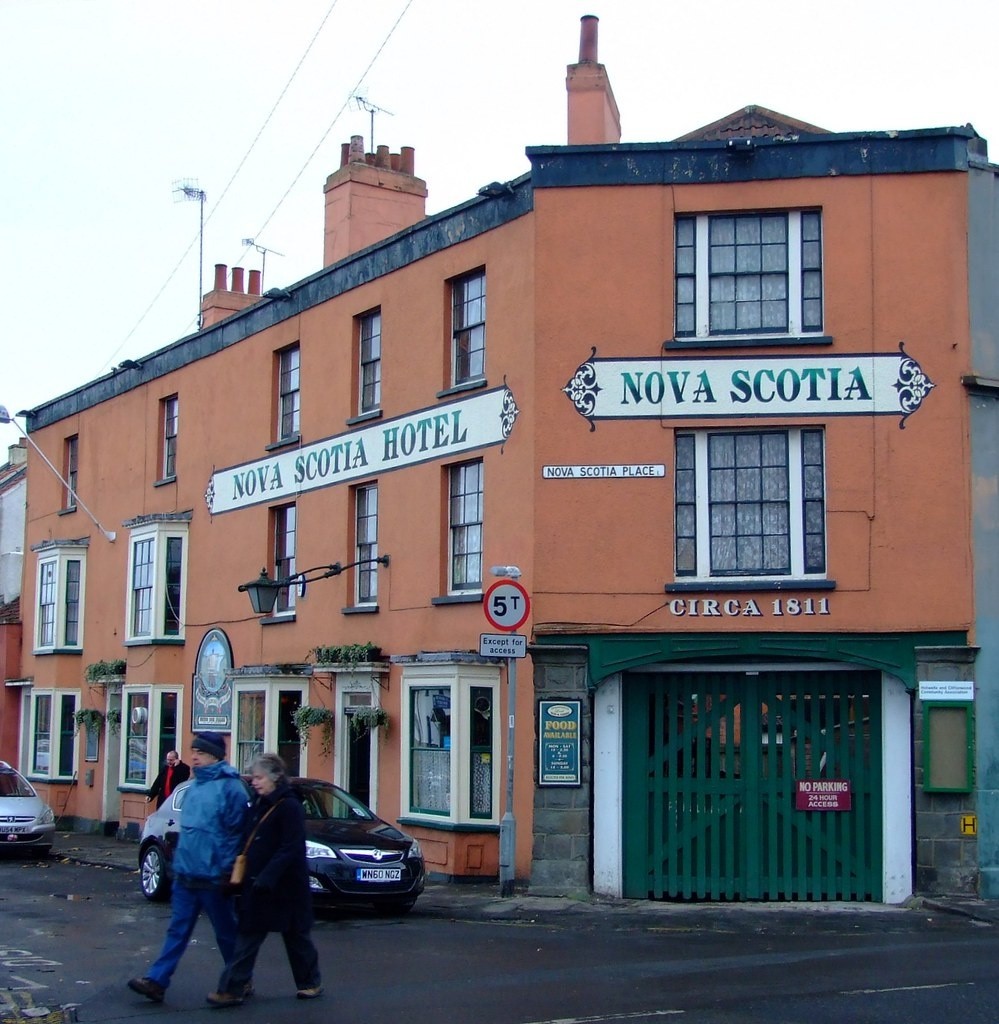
727;137;756;161
264;286;289;304
478;178;515;203
238;551;394;614
16;408;36;419
118;357;143;371
0;403;118;544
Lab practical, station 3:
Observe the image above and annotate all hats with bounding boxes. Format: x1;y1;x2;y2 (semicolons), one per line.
191;730;225;759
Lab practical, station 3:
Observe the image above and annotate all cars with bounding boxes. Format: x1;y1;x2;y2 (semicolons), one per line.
137;778;426;914
0;761;55;858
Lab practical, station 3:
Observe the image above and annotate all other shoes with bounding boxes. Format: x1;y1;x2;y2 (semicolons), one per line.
296;985;324;998
243;981;255;996
128;977;166;1002
207;992;242;1005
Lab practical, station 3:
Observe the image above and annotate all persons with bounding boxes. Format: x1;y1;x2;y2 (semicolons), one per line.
207;753;324;1006
144;750;190;811
125;732;255;1001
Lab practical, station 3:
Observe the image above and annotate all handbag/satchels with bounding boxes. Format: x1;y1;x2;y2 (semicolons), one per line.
230;855;247;885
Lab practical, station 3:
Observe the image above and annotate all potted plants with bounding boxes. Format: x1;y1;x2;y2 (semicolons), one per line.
293;706;338;765
106;708;120;734
353;708;391;738
71;707;102;738
302;642;387;674
84;659;126;693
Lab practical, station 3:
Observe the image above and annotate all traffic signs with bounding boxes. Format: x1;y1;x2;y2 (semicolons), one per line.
479;632;526;658
